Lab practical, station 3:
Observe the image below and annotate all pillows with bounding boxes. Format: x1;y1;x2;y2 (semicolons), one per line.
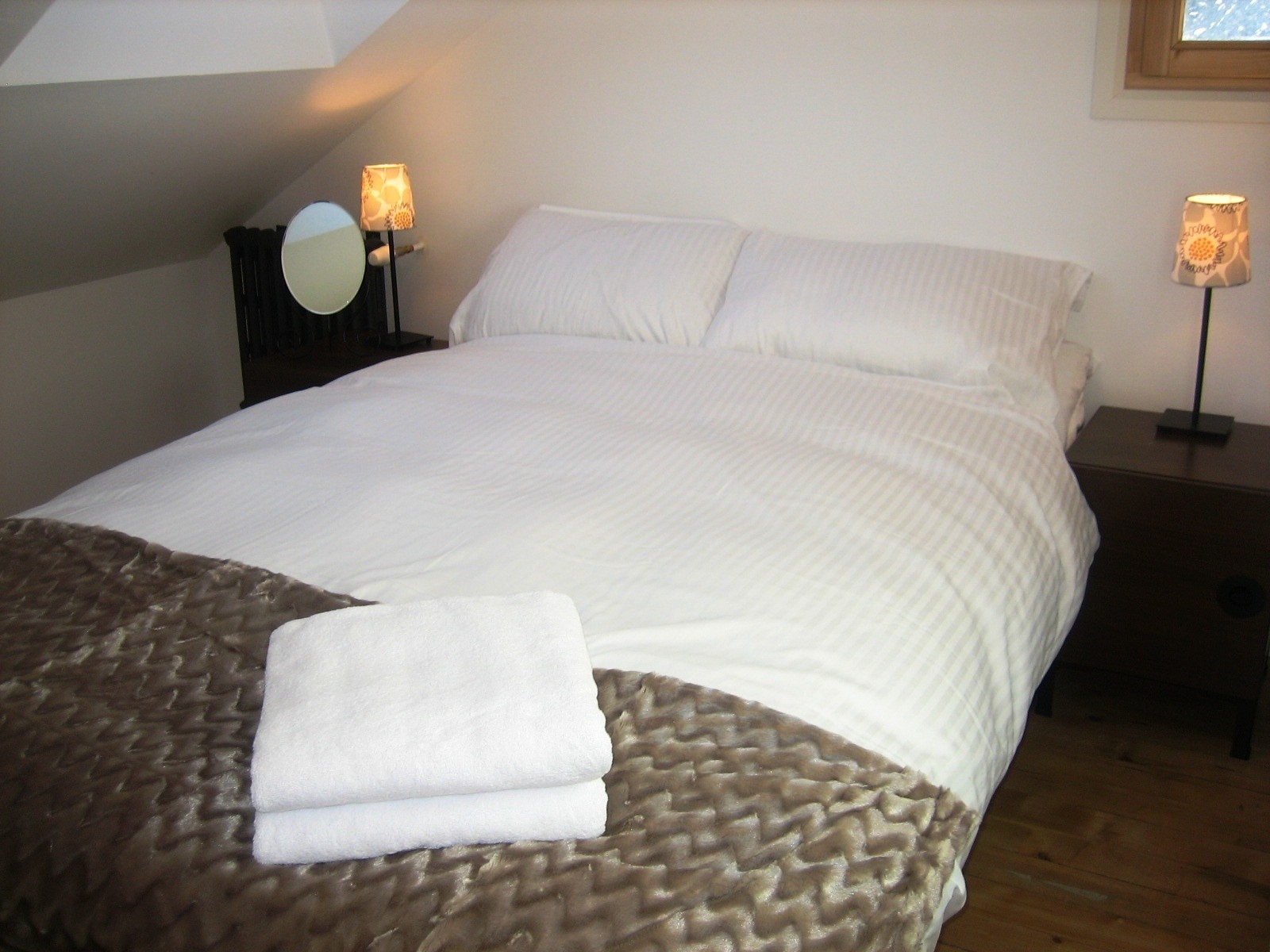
700;229;1094;428
450;204;751;349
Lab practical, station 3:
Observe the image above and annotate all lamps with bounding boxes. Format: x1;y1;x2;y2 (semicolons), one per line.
359;165;435;351
1155;192;1253;442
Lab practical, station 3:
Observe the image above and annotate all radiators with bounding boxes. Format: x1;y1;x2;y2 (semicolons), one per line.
223;226;389;406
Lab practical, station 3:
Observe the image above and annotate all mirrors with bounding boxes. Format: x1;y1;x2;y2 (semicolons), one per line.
278;200;367;370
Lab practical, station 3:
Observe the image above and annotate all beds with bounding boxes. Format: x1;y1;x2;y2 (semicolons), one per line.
0;204;1098;952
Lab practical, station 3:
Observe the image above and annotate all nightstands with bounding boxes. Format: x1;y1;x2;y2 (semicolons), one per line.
1064;406;1270;762
